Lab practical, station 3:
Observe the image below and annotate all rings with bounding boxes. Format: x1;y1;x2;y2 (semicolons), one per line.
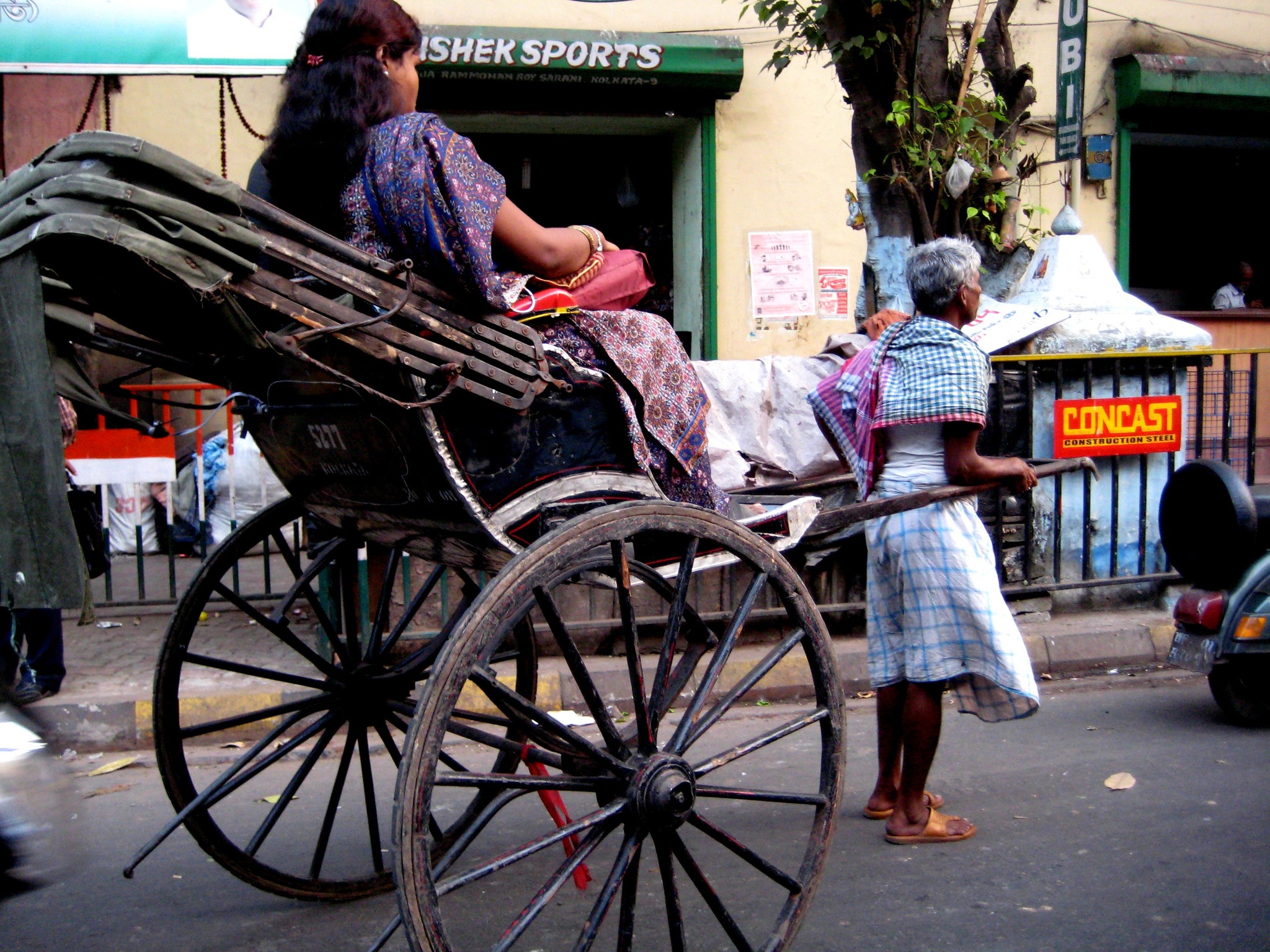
1028;486;1030;489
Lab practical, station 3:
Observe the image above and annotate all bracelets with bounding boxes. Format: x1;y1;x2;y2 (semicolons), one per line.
567;220;607;260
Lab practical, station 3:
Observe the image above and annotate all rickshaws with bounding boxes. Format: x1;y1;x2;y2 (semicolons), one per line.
0;131;1100;951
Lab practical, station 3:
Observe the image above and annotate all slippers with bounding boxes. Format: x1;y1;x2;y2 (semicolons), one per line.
886;806;977;845
861;788;944;820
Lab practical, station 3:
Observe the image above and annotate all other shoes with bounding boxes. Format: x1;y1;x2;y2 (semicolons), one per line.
724;495;767;520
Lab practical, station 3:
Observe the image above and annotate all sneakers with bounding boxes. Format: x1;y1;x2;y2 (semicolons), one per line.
0;663;52;706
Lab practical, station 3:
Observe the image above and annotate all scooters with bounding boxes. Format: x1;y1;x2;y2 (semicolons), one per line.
1157;461;1270;734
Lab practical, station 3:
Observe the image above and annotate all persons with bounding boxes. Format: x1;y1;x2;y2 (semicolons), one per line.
806;236;1044;845
241;0;726;589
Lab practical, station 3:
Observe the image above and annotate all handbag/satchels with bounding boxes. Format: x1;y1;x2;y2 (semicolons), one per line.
531;246;655;310
63;473;112;580
